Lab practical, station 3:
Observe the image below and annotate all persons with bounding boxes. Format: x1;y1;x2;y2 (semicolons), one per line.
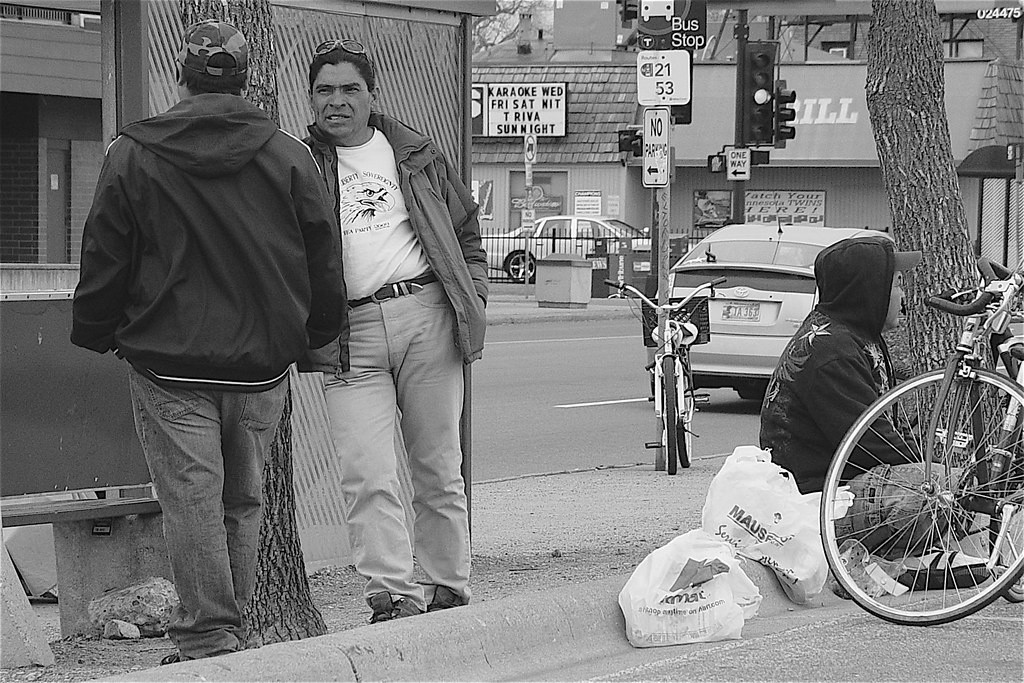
697;190;716;229
299;38;488;623
69;16;348;667
760;234;989;590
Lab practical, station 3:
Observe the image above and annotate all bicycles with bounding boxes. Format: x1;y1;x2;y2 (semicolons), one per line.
817;256;1024;626
603;275;729;476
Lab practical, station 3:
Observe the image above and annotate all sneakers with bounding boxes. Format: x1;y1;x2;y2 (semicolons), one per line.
369;591;423;625
426;584;468;612
888;546;992;589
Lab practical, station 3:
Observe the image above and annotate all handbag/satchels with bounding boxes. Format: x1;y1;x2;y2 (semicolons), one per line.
700;445;856;605
617;528;764;648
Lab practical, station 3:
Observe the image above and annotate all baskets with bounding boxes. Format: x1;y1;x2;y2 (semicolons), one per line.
641;296;711;346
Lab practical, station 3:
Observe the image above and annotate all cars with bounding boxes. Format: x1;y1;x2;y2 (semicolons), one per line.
478;212;650;283
649;222;899;401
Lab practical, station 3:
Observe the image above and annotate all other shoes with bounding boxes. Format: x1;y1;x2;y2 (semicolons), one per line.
159;649;230;666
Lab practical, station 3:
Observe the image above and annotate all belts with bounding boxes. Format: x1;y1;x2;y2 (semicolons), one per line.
347;270;438;310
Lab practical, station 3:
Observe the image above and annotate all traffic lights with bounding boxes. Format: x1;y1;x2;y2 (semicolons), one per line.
743;40;777;143
706;154;727;174
750;149;771;165
617;128;643;159
771;78;799;151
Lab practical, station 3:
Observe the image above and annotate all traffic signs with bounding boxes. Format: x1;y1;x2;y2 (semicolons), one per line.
727;148;752;182
641;107;672;188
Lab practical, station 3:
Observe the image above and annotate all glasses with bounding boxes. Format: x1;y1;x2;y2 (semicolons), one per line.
313;40;371;67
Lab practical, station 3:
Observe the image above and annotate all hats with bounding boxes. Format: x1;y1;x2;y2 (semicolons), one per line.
893;252;922;271
175;20;249;76
